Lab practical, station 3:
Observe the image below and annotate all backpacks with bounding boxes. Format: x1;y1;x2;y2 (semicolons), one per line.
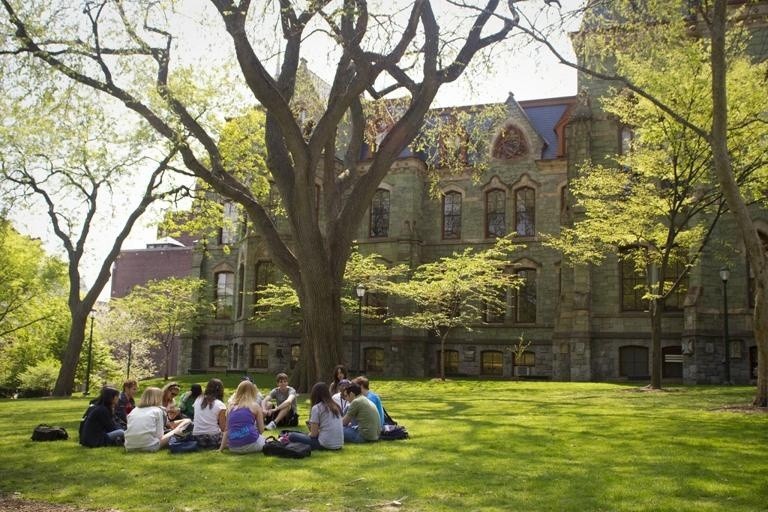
32;424;67;441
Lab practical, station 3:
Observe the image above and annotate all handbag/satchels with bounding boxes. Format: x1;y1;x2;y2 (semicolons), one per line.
168;430;198;453
263;436;311;458
382;424;407;440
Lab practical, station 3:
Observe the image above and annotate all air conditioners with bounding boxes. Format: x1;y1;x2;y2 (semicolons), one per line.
515;367;530;376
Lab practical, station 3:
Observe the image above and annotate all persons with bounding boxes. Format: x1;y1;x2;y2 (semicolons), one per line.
261;372;298;432
287;367;385;451
80;378;267;452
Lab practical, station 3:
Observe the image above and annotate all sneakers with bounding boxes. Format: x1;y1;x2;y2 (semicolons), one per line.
265;421;277;430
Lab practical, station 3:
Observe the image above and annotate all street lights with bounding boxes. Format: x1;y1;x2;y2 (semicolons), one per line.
716;265;733;388
353;283;366;378
82;306;97;394
